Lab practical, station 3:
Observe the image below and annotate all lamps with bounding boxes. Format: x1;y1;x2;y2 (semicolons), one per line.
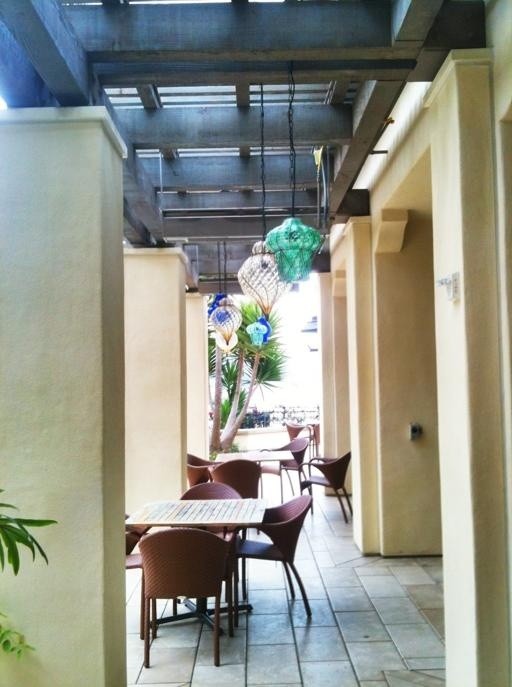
209;63;326;350
